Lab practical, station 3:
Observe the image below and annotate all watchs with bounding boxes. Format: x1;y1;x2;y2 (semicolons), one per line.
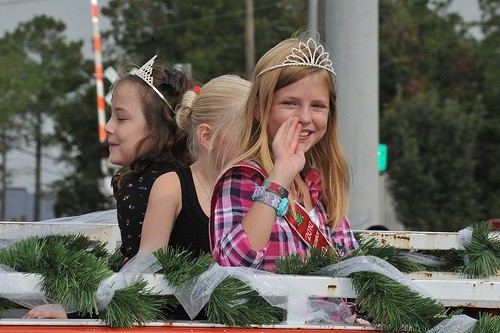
252;188;290;217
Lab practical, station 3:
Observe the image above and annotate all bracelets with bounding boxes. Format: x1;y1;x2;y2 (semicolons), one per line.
259;177;290;198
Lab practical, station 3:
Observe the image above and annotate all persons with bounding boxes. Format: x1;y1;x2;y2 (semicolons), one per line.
137;71;264;278
210;28;370;283
22;61;192;320
